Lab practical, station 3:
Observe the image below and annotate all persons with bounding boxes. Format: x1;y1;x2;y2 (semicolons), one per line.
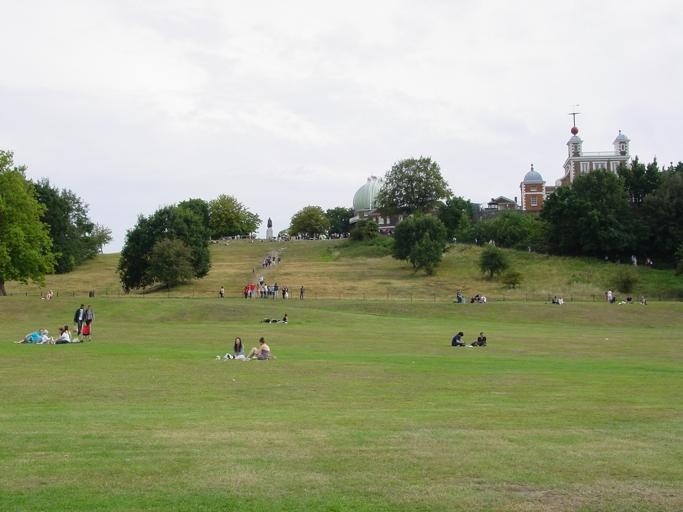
604;288;647;306
453;236;457;244
223;337;245;359
378;228;393;237
247;337;271;360
262;255;281;269
14;289;94;344
210;230;351;246
244;281;288;299
300;286;304;300
452;332;469;347
456;289;486;304
221;286;224;298
632;254;637;266
283;313;288;322
488;239;496;246
470;332;486;347
552;295;564;305
